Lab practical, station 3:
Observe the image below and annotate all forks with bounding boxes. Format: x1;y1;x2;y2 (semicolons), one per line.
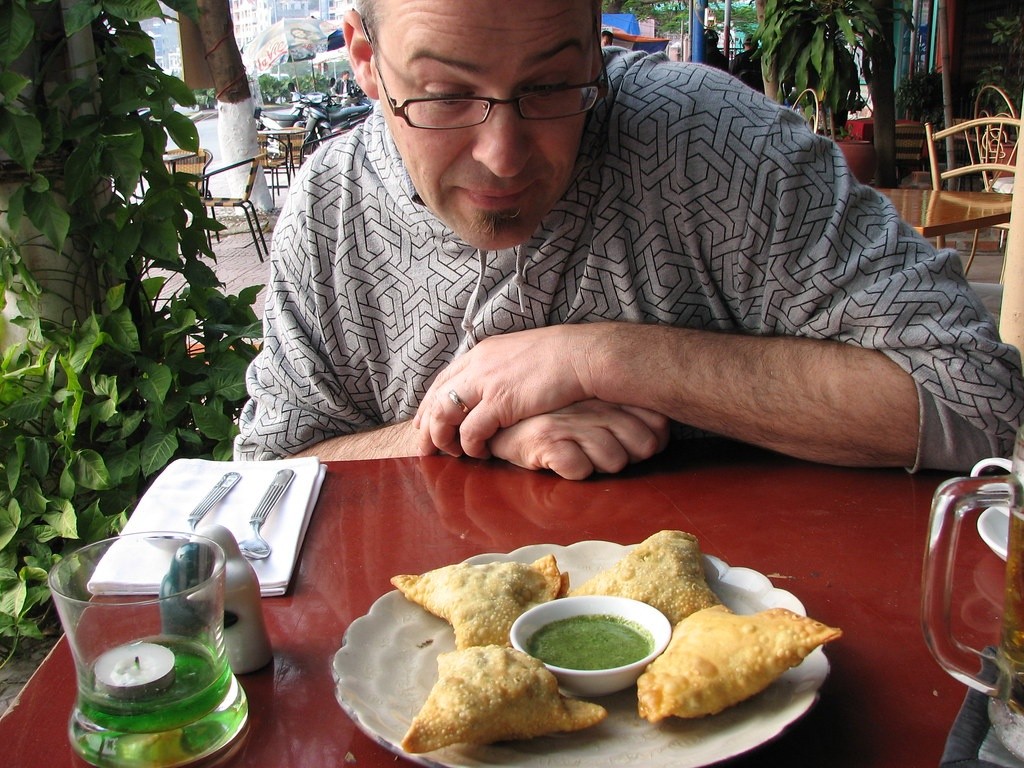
236;469;296;561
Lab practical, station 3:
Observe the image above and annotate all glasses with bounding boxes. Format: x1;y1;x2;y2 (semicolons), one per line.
352;0;610;130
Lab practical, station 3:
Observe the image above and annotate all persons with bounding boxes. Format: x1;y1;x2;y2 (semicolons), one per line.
730;33;765;94
601;31;613;46
291;28;325;43
234;1;1024;478
331;72;354;105
704;29;729;73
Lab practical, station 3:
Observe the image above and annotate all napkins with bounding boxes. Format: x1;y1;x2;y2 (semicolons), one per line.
86;458;328;597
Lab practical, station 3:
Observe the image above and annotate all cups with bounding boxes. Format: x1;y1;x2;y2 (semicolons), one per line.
48;529;249;768
919;424;1024;762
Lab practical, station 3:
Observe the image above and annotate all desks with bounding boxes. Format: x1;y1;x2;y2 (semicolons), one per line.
844;116;929;161
943;134;1018;192
0;456;1005;768
875;187;1014;238
140;153;197;198
256;128;308;179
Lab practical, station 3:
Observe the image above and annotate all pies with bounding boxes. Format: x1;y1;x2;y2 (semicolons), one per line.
400;645;608;753
388;555;571;647
567;529;720;625
636;604;843;720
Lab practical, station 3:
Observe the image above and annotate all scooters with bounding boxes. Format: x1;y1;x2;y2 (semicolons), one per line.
254;77;373;154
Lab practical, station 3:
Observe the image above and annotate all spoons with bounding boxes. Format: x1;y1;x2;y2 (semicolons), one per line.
143;470;242;551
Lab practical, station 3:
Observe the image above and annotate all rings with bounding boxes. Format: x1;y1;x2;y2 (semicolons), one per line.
448;389;470;414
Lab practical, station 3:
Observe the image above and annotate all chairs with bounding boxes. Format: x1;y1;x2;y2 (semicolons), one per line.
165;149;221;243
195;153;270;264
260;126;353;208
923;117;1020;249
894;125;925;183
964;81;1020;282
934;118;975;160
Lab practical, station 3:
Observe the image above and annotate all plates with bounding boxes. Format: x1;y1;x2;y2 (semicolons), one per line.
330;539;831;768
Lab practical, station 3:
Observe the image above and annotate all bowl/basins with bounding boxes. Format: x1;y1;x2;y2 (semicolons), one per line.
510;595;675;699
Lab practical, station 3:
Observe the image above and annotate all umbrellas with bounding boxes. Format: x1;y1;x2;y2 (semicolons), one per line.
241;16;347;90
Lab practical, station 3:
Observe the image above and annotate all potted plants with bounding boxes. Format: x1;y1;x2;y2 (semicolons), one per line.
745;0;917;185
970;14;1024;179
